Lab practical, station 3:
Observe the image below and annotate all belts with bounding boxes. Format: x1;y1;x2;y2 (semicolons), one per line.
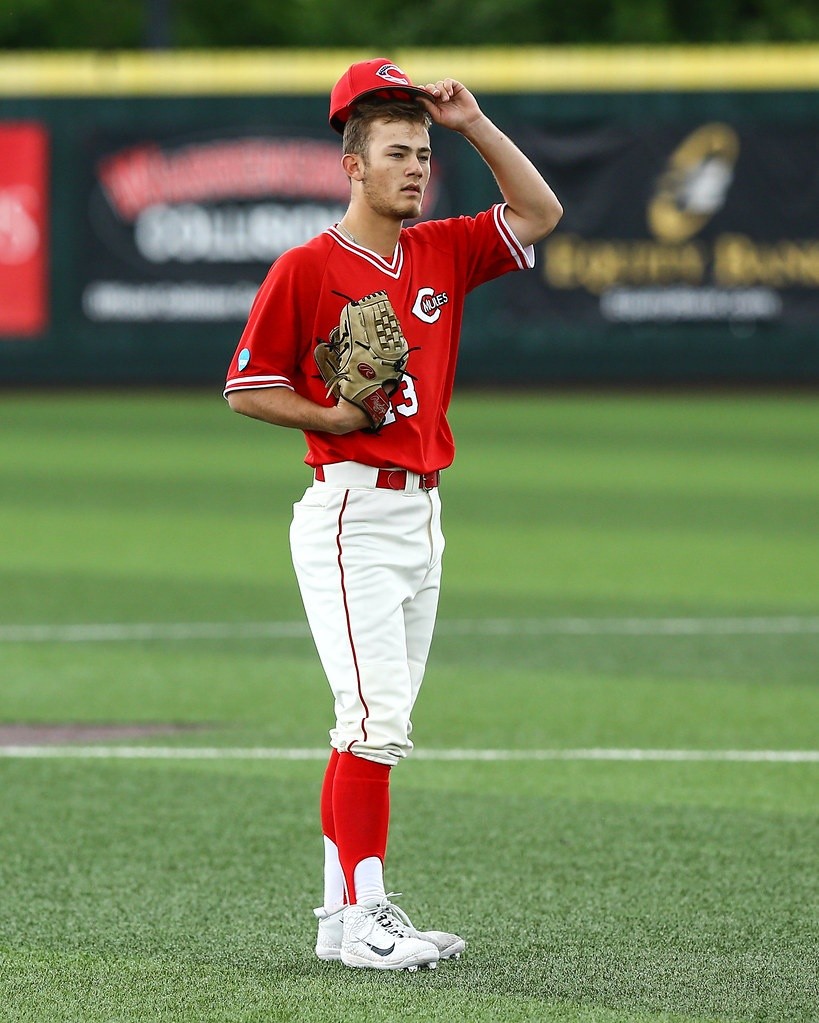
314;465;440;492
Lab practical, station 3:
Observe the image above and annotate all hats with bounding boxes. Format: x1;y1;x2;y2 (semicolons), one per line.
328;58;436;135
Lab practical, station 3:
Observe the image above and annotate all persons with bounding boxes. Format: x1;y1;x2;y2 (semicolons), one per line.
221;58;563;974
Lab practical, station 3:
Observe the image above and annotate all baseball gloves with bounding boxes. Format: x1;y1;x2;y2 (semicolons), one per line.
310;288;425;434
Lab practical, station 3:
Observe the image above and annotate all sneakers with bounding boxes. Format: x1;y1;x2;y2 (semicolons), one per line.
313;905;467;960
340;893;439;972
